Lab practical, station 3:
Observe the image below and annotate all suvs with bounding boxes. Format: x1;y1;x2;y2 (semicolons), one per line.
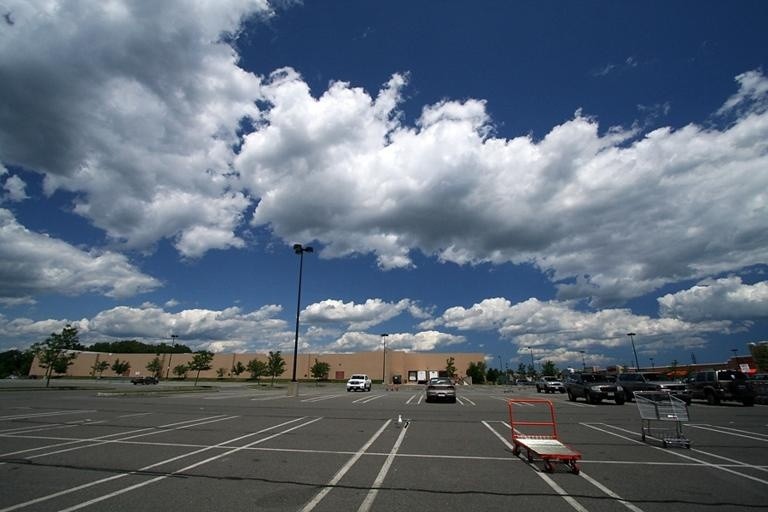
535;369;768;404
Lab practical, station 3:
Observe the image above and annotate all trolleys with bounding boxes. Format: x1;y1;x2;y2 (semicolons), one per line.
633;389;690;449
507;399;583;474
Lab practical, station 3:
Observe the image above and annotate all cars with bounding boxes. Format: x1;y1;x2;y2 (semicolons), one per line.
517;379;528;385
427;377;456;401
346;374;371;391
132;376;159;384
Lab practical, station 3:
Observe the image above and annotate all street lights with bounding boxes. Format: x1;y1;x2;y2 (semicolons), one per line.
290;243;313;382
166;334;179;379
381;333;388;380
627;332;639;368
